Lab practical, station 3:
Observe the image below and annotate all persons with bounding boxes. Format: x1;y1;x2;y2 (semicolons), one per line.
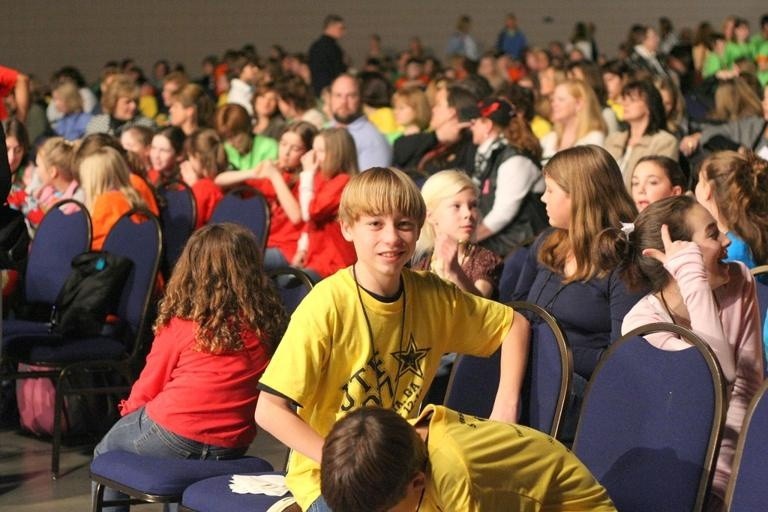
0;5;768;512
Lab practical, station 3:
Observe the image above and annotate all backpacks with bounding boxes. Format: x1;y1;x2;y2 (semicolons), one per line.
44;249;136;352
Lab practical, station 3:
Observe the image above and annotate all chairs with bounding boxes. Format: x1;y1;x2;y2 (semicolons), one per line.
2;206;162;481
722;377;767;511
179;300;574;512
169;182;271;265
87;267;317;512
746;264;768;342
1;196;95;384
568;324;731;510
147;177;197;275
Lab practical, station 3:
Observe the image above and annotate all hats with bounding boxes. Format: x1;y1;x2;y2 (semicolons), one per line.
460;96;512;127
